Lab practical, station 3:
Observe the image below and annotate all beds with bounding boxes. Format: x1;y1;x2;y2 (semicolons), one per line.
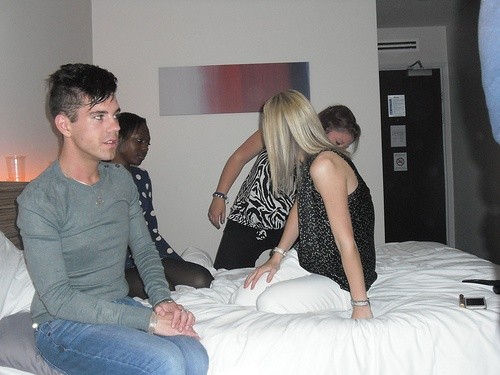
0;180;500;375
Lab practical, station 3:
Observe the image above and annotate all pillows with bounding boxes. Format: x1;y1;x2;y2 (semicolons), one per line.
0;230;23;313
0;312;66;375
0;250;35;319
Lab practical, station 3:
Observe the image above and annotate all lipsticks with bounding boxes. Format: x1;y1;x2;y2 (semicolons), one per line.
459;293;465;306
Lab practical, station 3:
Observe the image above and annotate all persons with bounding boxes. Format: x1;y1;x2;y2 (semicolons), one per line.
15;63;209;375
229;88;377;319
100;112;214;299
207;106;360;270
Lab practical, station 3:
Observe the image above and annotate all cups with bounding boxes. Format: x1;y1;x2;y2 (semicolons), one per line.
5;154;25;181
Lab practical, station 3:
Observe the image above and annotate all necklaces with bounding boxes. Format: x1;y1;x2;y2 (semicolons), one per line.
63;171;105;206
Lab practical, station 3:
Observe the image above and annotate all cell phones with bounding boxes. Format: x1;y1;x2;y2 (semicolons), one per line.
465;297;487;309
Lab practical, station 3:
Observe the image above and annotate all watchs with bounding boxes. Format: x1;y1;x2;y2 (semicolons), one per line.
269;247;286;257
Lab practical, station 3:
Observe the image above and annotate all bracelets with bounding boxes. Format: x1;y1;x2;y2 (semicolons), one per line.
212;192;229;204
351;298;369;306
